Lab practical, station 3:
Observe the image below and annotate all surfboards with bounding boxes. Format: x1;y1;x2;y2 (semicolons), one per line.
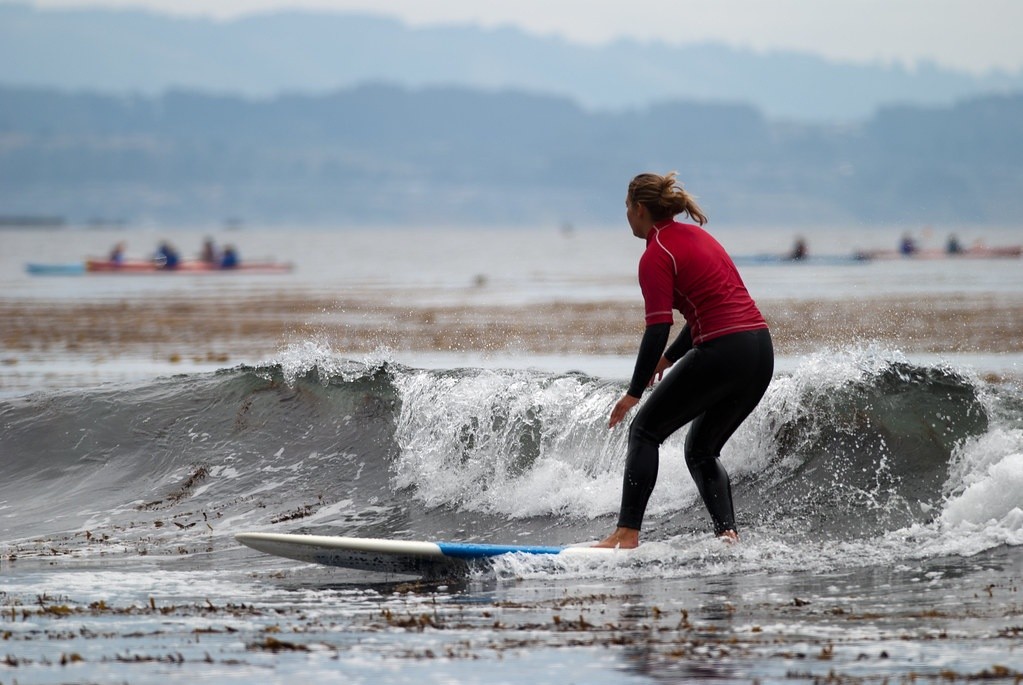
232;529;660;578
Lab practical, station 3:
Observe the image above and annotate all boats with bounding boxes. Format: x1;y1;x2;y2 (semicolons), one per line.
85;256;297;276
25;260;87;277
729;246;1023;264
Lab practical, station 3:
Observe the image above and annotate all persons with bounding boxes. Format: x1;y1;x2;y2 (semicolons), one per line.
945;234;960;253
790;238;809;259
220;245;239;267
589;172;774;549
110;243;126;262
897;231;915;254
197;237;214;260
156;239;179;266
